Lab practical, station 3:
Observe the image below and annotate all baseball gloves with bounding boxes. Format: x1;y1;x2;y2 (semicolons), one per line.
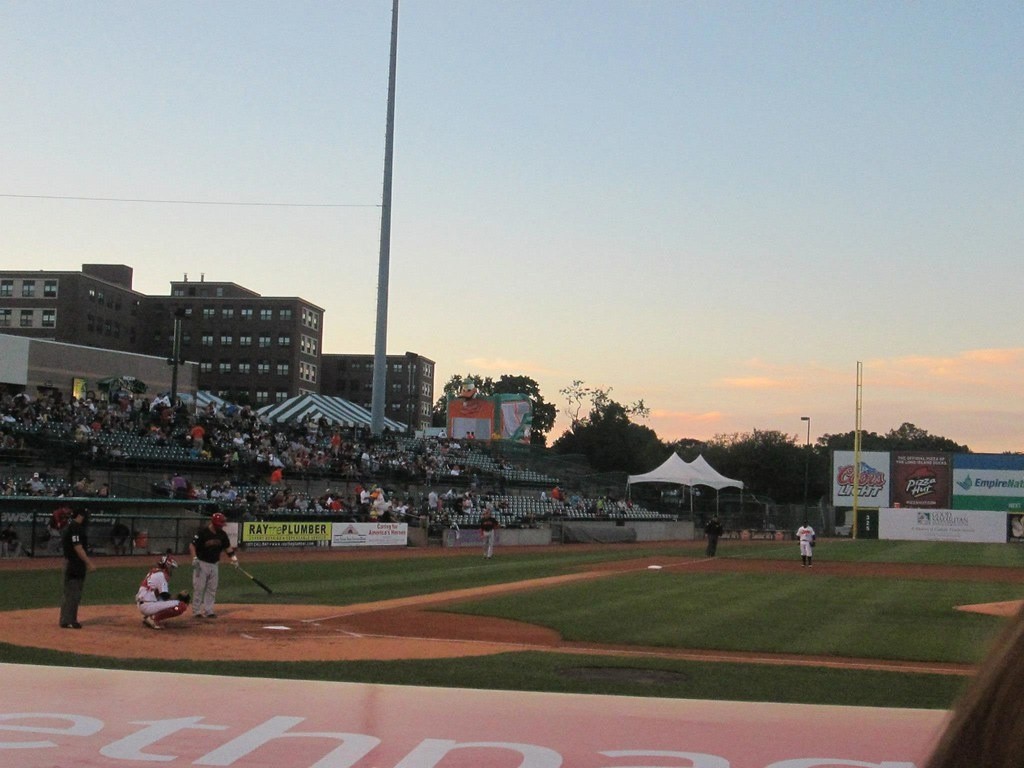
177;593;191;606
810;541;816;547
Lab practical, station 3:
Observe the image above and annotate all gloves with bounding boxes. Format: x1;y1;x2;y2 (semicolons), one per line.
191;557;199;569
230;556;238;568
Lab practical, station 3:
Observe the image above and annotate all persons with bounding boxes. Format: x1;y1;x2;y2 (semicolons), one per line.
795;518;816;568
135;553;187;630
703;513;723;558
58;506;98;629
189;512;239;622
0;381;635;558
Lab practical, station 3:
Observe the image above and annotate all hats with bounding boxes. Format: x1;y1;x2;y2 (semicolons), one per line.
34;472;39;478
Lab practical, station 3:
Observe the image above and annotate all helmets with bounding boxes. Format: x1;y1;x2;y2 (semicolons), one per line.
157;556;178;569
211;513;226;526
72;502;88;516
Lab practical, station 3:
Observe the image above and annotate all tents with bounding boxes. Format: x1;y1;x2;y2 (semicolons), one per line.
624;450;747;522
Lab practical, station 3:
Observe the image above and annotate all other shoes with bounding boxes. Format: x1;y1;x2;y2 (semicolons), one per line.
143;615;164;629
205;614;216;619
194;614;204;620
61;623;80;629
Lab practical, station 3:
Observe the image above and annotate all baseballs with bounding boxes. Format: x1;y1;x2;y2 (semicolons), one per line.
237;566;274;594
